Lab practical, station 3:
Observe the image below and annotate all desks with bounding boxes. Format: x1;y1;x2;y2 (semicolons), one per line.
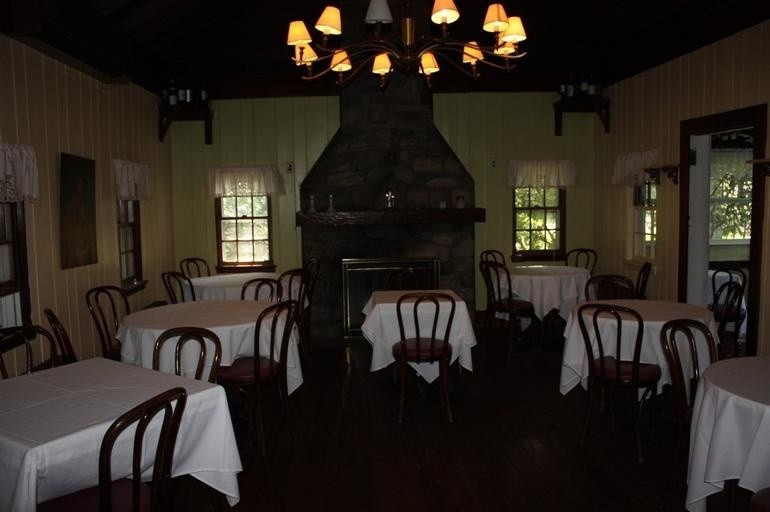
683;355;770;511
358;287;478;404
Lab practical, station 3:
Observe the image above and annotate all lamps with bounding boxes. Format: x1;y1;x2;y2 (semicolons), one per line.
287;0;527;89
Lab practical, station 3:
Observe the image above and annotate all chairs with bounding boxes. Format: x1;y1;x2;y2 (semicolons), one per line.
479;247;747;465
390;265;432;289
1;257;321;511
393;292;456;424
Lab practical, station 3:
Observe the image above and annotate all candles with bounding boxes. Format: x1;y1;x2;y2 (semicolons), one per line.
329;194;333;210
310;194;314;212
